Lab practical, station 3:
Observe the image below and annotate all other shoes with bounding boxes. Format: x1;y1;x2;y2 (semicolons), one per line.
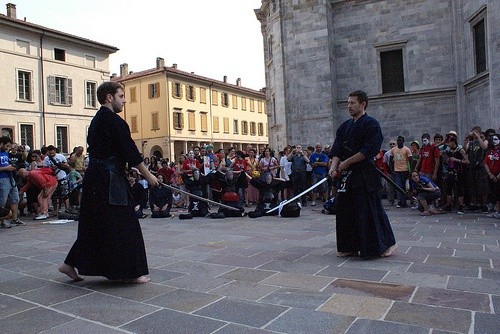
458;208;464;214
486;212;494;217
0;220;11;228
48;209;53;213
34;213;50;220
465;203;489;214
419;211;430;216
388;201;394;205
19;212;26;217
494;212;500;218
10;219;25;225
397;204;401;208
311;201;316;206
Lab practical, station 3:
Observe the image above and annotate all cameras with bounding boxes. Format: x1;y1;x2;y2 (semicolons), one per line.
469;132;475;136
12;163;18;169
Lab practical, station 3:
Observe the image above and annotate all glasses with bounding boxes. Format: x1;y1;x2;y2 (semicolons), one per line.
389;143;395;145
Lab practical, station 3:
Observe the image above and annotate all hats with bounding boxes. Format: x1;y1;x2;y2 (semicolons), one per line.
448;130;457;137
411;140;420;149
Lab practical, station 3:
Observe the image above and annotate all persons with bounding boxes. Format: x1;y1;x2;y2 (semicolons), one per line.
326;90;401;257
0;126;500;228
55;81;152;284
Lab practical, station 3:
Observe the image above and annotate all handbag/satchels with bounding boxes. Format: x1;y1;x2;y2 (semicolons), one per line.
279;202;301;217
182;173;194;186
